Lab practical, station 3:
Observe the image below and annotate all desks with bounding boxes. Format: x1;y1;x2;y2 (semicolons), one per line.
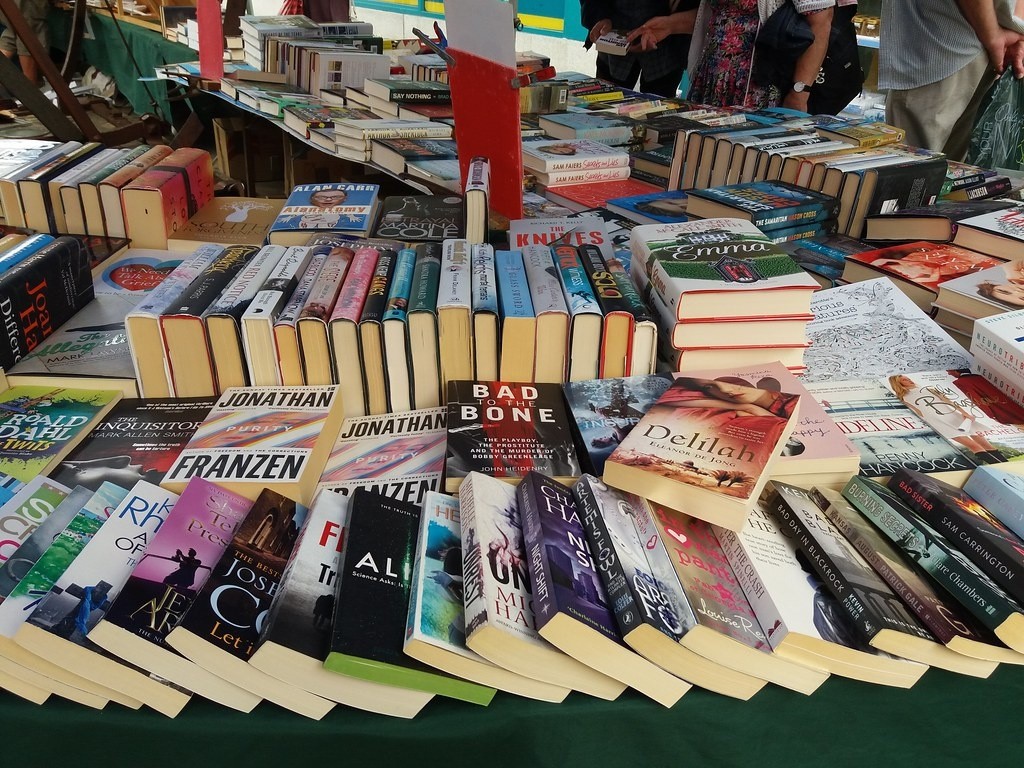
49;7;199;124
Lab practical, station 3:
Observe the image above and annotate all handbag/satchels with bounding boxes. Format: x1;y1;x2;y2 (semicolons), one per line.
962;64;1024;170
755;3;875;116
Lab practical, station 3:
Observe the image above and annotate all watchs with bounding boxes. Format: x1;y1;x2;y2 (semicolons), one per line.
791;81;813;93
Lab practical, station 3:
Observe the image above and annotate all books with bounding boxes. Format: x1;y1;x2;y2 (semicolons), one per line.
0;0;1024;719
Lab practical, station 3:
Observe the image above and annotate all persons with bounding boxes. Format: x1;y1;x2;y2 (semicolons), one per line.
625;0;859;114
877;0;1024;166
309;188;348;208
655;377;800;459
579;0;704;100
869;244;987;283
0;0;49;88
888;374;1008;466
946;368;1023;432
975;257;1024;311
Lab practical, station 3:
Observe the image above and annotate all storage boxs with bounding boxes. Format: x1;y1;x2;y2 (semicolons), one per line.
212;117;283;180
